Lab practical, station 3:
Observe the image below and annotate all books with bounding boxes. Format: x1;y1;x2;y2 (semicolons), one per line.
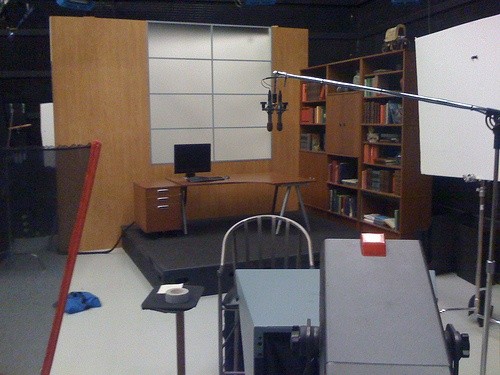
362;210;400;231
328;189;356;218
363;77;377;97
301;106;323;124
363;101;387;125
300;132;326;152
327;160;358;186
361;169;400;196
302;82;326;101
362;145;399;166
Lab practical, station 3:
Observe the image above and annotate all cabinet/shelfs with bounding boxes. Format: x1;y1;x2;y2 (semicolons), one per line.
132;178;182;235
301;49;437;238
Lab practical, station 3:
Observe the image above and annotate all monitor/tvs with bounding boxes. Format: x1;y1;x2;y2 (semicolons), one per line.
173;144;210;176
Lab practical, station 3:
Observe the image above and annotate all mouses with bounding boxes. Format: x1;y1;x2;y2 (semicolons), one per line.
223;177;229;179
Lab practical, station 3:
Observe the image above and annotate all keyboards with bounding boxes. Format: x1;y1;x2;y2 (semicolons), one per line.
189;176;224;182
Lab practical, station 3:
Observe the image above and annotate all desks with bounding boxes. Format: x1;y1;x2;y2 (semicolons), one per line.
168;172;319;236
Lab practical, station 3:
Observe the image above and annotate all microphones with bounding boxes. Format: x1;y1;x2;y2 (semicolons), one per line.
267;90;273;130
277;91;282;131
